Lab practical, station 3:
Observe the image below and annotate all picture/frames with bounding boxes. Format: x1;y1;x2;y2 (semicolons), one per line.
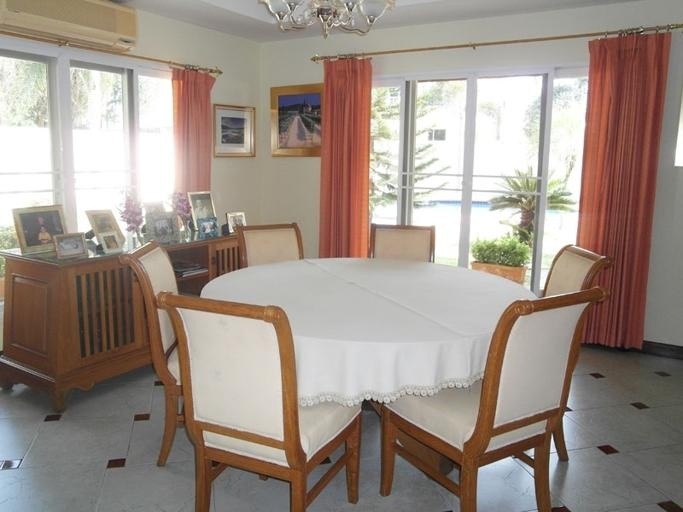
226;211;246;235
213;103;256;157
53;232;90;259
196;218;220;240
269;83;321;157
188;191;218;231
98;232;121;253
146;213;178;244
13;205;67;257
86;209;125;246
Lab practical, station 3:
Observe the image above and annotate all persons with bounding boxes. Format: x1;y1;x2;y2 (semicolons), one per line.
37;216;50;243
194;199;210;220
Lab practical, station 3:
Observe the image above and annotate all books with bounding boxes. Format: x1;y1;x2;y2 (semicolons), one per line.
173;265;209;278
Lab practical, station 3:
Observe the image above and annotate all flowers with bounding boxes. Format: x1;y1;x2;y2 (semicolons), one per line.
120;196;143;233
173;190;191;219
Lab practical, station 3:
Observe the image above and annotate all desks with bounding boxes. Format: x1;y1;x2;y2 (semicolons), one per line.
0;235;239;412
198;259;540;482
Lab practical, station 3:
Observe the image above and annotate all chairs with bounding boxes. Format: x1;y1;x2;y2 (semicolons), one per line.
544;245;613;303
119;239;186;466
369;223;435;263
157;290;362;512
235;222;305;268
380;286;611;512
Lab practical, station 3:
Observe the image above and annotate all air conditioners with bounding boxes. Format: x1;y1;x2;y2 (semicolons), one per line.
1;0;138;56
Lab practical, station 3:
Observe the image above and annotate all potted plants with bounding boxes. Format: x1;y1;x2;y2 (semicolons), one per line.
0;226;20;302
469;235;531;284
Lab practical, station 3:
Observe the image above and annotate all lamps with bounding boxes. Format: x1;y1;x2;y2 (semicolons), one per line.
260;0;395;39
